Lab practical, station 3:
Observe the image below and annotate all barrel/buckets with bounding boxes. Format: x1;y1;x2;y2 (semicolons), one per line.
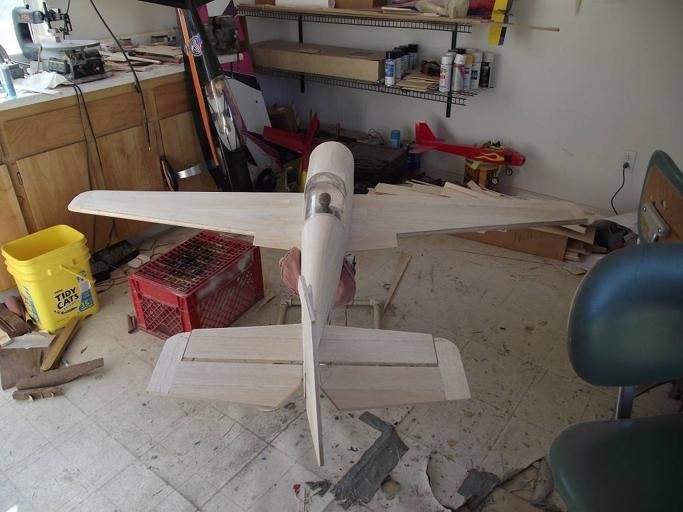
1;224;101;332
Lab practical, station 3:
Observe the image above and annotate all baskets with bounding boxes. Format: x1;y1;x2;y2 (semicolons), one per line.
129;231;264;341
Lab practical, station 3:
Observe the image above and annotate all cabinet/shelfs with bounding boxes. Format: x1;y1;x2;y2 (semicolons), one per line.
237;8;493;118
0;52;243;291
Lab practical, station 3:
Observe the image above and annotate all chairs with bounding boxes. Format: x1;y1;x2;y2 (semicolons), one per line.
550;242;683;512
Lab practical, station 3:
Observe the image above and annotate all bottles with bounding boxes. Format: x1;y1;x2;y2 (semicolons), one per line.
438;47;496;93
383;43;419;87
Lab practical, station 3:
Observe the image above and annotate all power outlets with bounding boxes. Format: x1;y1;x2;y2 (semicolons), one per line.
616;151;637;173
